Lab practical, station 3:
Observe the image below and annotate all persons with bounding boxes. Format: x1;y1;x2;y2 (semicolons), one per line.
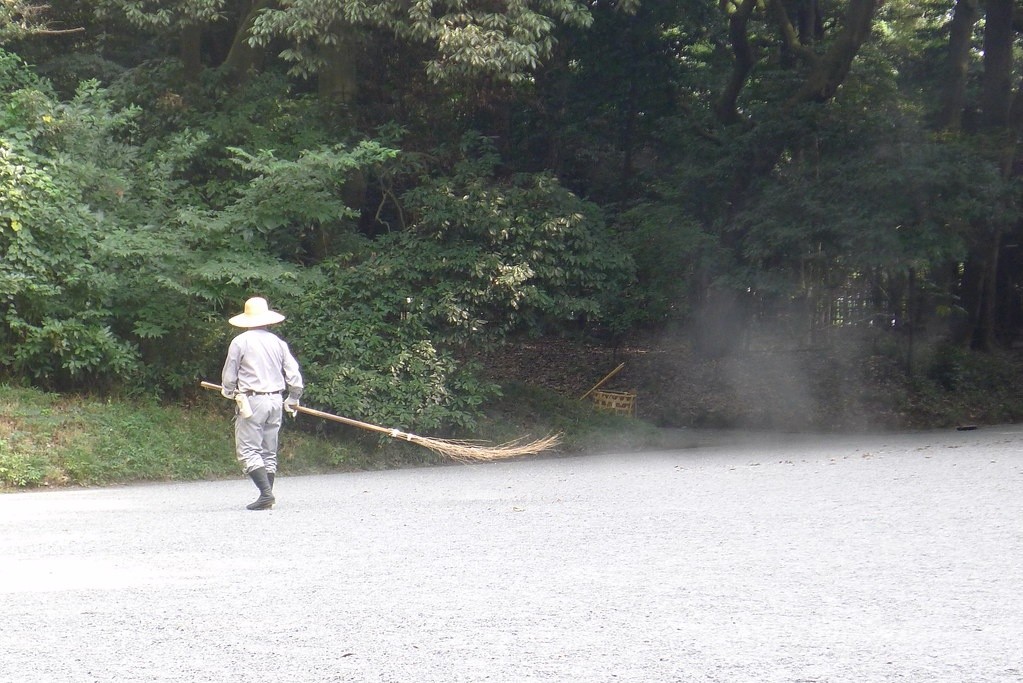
220;297;304;510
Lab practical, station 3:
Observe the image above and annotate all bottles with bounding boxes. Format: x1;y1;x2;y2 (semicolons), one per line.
234;389;253;419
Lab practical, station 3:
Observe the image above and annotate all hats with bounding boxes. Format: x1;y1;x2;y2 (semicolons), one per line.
229;297;286;327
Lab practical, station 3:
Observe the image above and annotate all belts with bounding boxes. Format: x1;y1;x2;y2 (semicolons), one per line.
241;390;279;396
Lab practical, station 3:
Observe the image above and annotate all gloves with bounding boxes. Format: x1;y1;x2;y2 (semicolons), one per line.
284;397;299;418
221;389;234;400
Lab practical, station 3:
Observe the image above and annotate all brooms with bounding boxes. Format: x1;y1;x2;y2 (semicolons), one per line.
200;380;566;467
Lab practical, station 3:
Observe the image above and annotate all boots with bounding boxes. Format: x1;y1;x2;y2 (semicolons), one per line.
247;473;275;508
249;467;275;509
